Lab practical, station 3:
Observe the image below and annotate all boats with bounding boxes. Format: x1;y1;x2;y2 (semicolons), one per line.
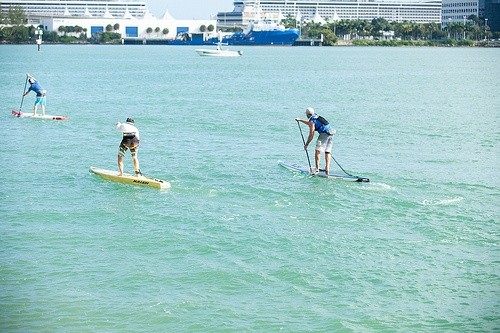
196;47;242;57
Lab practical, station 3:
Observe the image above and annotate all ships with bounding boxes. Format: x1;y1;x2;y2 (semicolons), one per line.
168;25;302;47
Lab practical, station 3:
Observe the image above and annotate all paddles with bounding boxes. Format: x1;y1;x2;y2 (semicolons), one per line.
17;75;29;117
297;120;315;178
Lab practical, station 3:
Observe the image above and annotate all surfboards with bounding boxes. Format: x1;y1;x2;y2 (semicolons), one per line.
11;110;67;120
89;166;171;189
278;159;370;184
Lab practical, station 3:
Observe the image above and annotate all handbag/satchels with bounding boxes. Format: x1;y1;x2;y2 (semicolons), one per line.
317;115;329;125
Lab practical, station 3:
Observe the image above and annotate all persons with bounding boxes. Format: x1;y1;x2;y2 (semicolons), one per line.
22;73;46;116
295;107;334;175
116;118;142;177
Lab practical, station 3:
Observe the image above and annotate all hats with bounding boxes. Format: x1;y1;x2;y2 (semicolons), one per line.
304;107;314;115
28;78;34;83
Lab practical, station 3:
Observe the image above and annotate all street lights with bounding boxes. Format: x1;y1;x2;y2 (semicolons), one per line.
484;18;489;41
